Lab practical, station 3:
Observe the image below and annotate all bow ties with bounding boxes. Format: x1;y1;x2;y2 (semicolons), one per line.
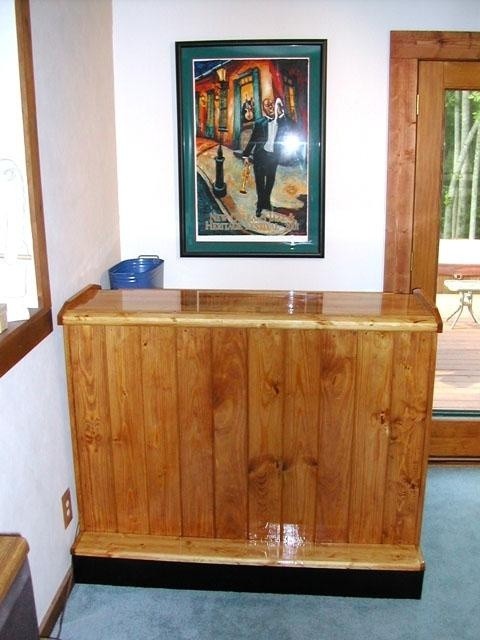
268;118;274;122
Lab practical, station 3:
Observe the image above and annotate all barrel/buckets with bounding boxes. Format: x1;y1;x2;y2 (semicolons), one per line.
107;253;164;287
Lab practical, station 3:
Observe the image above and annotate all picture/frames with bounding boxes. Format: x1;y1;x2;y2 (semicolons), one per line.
175;39;327;258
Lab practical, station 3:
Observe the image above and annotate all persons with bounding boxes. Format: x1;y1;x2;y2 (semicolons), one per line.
242;99;295;218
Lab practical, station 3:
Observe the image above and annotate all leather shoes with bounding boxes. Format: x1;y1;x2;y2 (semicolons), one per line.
256;202;271;217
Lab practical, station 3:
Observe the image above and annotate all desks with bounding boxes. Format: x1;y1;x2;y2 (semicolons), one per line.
443;279;480;329
